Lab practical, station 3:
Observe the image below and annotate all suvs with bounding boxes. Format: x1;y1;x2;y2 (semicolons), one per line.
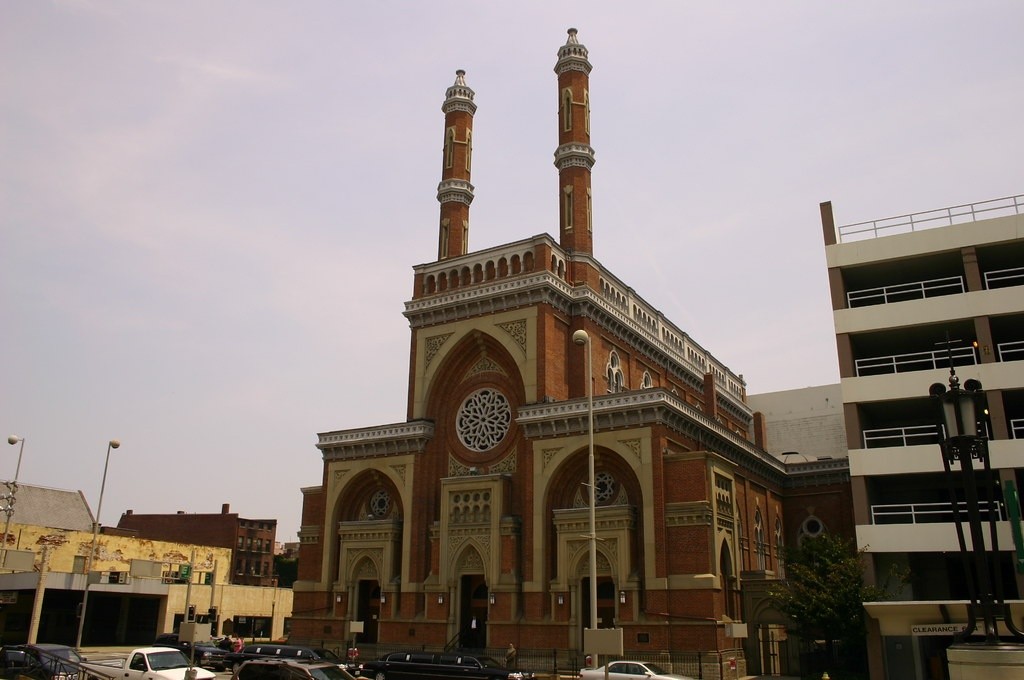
229;656;368;680
151;632;230;673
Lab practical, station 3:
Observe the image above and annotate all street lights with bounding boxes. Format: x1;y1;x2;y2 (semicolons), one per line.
929;341;1024;649
573;330;598;669
0;435;26;572
75;439;120;651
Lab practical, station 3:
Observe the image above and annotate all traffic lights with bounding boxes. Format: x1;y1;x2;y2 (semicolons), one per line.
207;605;219;623
188;606;197;622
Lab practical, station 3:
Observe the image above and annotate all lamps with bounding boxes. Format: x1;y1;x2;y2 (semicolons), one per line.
438;594;443;604
469;467;478;476
620;592;625;603
336;593;341;602
558;595;563;604
489;594;495;604
380;595;385;604
367;515;374;520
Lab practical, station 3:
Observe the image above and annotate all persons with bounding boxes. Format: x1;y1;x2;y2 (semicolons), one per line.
468;613;481;648
218;634;245;654
506;641;516;669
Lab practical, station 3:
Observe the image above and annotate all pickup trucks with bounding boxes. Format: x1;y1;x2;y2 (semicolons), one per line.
77;646;216;680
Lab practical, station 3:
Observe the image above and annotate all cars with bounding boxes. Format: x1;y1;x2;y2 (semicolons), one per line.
578;658;695;680
223;643;363;678
360;649;535;680
0;644;98;680
